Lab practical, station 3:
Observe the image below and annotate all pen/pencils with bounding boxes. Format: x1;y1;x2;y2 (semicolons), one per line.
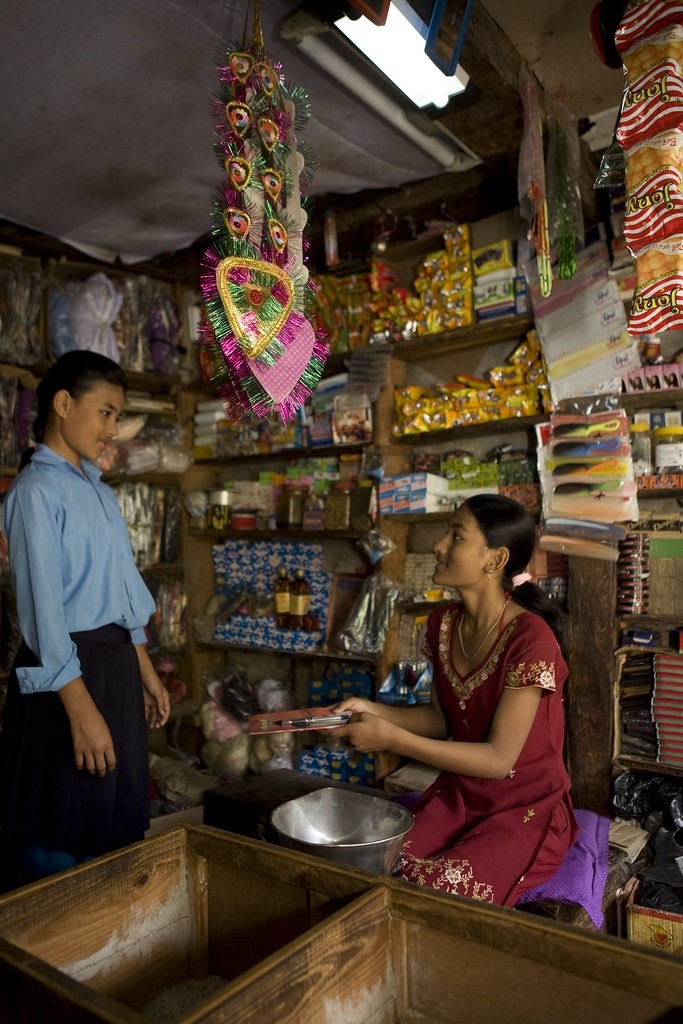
273;714;349;726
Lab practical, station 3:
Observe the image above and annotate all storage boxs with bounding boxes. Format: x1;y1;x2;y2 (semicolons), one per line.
625;877;683;962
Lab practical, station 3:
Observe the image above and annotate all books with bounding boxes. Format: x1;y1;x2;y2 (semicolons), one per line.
248;707;344;735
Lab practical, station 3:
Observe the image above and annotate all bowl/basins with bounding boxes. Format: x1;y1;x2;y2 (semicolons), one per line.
271;787;414;876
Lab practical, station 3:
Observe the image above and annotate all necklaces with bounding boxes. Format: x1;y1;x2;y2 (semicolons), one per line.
459;591;512;659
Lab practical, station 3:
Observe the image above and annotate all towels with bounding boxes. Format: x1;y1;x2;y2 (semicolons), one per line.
194;400;227;446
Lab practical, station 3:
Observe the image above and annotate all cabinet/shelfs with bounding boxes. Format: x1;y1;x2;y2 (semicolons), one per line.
0;203;682;877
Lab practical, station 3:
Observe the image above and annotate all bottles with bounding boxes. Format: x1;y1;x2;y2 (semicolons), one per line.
290;570;310;629
274;567;291;628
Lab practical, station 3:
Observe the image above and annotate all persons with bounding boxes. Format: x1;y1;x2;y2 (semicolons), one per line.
0;351;170;893
316;493;580;909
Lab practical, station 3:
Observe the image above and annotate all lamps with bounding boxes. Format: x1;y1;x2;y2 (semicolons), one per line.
274;9;485;173
